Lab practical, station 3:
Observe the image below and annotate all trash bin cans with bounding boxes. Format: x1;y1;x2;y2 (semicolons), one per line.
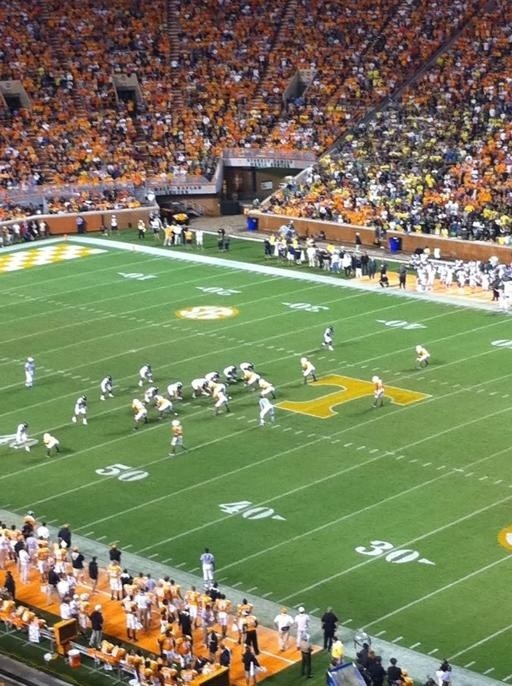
390;237;400;252
247;217;257;230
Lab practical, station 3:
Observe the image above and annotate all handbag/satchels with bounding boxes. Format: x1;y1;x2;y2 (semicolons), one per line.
281;626;290;632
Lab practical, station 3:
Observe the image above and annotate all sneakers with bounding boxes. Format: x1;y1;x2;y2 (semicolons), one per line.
16;342;430;459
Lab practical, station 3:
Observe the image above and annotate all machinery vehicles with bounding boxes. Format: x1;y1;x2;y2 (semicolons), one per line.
161;202;190;226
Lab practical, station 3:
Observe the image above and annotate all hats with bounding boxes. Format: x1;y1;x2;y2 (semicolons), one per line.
299;607;304;613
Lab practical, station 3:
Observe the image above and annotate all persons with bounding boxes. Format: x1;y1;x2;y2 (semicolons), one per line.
320;325;335;355
414;343;432;366
300;357;318;386
24;356;38;390
